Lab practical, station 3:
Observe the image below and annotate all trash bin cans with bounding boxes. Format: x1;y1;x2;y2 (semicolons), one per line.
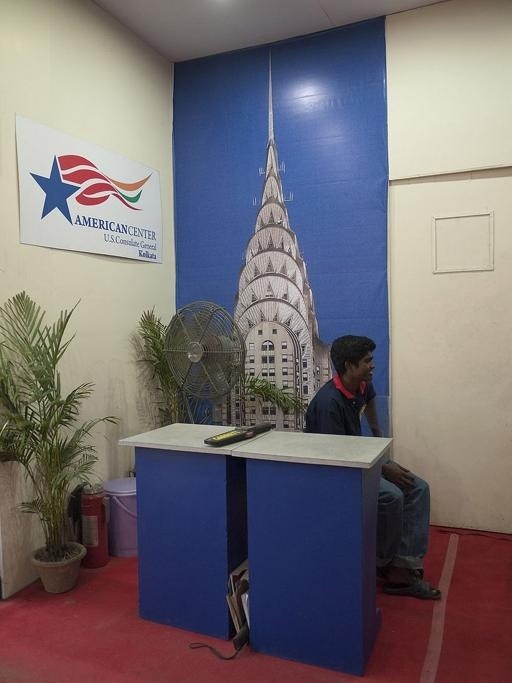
109;478;138;558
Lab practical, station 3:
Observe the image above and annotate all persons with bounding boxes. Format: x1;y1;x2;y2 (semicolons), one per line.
309;333;444;602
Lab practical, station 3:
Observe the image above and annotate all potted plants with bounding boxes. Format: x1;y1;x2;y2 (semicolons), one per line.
0;290;121;595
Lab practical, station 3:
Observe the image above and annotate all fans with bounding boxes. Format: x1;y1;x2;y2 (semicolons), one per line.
162;300;247;428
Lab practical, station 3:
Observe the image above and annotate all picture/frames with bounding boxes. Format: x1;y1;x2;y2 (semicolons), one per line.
430;208;495;274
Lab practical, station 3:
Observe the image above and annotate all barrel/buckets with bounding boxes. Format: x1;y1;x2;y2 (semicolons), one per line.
105;477;140;559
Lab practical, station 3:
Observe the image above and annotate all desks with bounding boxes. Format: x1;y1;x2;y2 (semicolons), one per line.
118;423;274;640
230;429;393;678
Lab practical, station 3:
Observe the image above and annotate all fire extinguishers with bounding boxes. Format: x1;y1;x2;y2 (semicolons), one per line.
82;483;110;567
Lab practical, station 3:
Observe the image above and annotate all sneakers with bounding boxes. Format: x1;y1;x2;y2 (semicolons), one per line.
376;568;441;599
